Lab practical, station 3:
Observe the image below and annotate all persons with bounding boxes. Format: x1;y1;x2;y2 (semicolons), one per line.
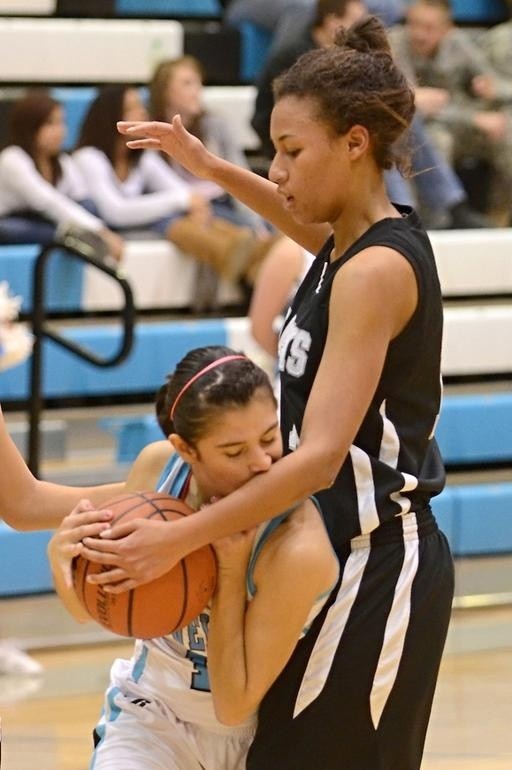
0;404;127;533
79;15;455;768
45;343;342;769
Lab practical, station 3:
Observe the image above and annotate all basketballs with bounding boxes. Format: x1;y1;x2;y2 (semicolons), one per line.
70;491;218;640
